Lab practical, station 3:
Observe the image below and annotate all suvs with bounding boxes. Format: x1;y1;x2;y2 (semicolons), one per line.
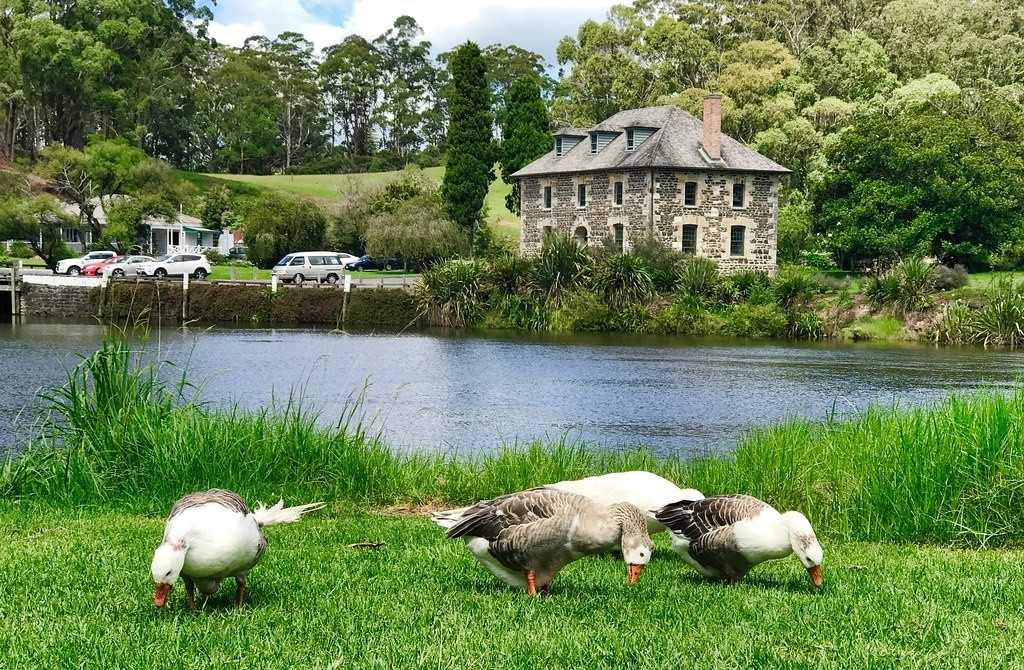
136;252;213;279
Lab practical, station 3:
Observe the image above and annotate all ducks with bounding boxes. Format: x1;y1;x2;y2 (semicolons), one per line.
650;494;823;588
150;488;327;613
431;469;706;596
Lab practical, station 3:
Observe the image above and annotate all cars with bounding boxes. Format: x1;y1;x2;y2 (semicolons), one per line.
82;254;125;277
337;253;359;266
103;255;156;278
345;255;398;272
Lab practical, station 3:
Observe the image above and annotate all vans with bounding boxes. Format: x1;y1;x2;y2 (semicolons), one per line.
229;246;249;259
270;251;344;284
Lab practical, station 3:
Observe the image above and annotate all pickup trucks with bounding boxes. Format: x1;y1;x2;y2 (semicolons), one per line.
55;250;117;277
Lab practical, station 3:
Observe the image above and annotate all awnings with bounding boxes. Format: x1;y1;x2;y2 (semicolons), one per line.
184;226;222;237
